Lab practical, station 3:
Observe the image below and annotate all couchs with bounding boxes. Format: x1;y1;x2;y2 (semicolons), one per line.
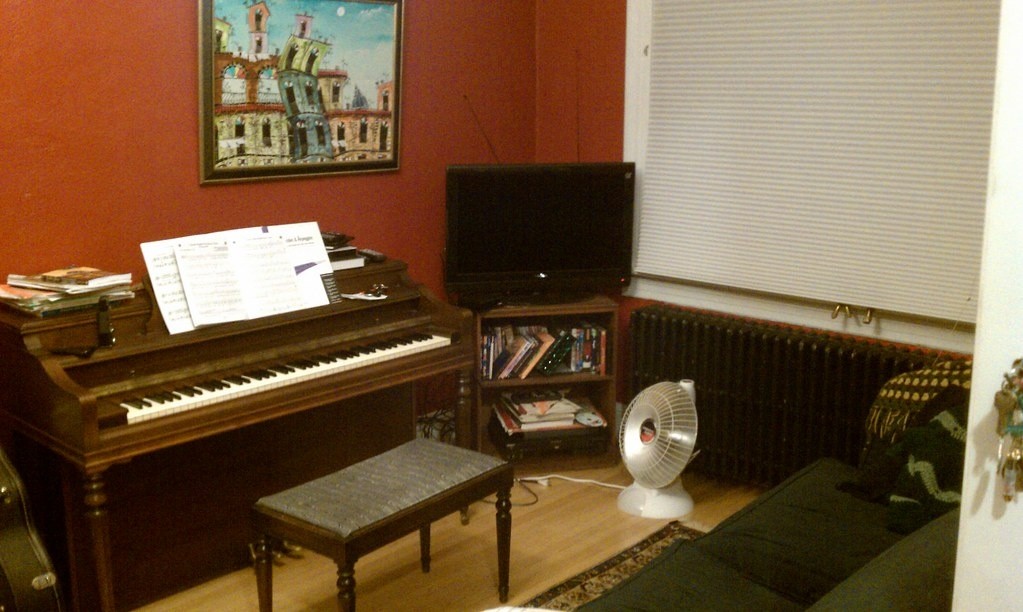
572;458;962;612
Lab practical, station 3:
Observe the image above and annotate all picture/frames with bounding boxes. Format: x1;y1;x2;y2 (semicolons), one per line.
194;0;406;185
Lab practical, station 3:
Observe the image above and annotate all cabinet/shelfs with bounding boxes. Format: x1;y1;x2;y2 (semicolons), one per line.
476;296;620;469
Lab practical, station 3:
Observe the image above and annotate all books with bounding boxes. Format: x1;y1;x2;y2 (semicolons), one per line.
139;219;367;337
0;265;137;317
493;385;609;436
480;320;608;379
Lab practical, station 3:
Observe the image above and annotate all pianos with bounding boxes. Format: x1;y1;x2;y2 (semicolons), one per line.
0;252;475;611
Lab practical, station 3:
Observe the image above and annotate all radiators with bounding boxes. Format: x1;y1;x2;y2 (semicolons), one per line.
622;303;965;491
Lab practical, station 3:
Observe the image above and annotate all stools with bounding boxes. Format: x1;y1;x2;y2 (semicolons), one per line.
249;437;513;611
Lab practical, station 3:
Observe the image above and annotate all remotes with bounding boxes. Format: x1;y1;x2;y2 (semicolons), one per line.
359;248;388;263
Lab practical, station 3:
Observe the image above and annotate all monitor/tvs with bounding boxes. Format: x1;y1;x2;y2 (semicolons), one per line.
445;161;635;294
